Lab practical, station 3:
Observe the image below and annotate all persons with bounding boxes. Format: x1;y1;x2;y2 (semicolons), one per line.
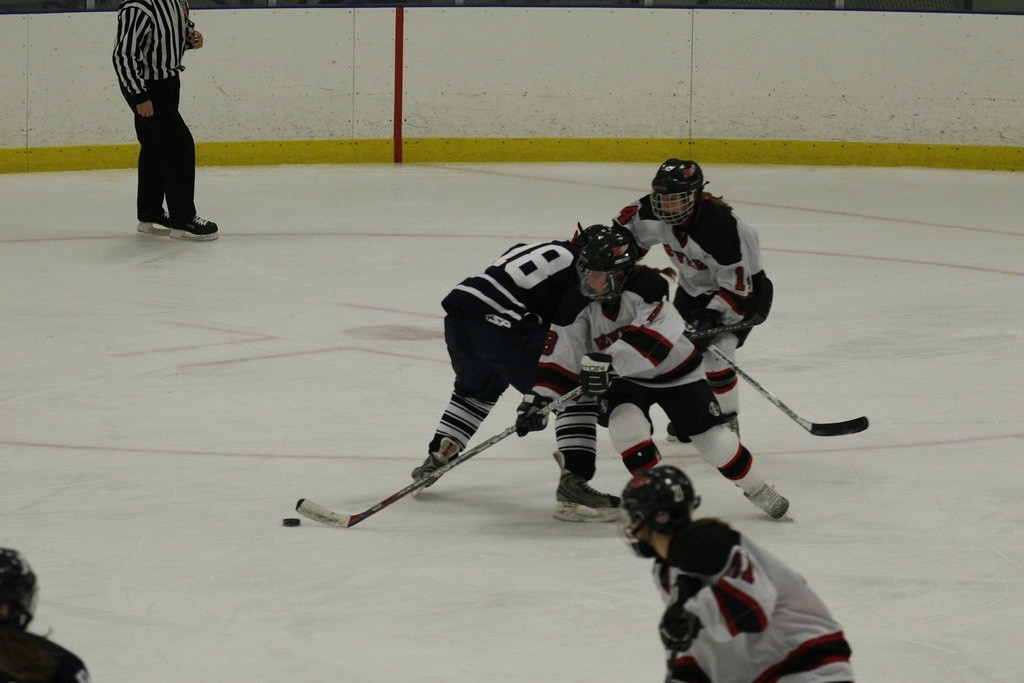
574;229;792;522
609;158;774;438
622;465;854;683
0;547;91;683
113;0;219;240
412;225;622;524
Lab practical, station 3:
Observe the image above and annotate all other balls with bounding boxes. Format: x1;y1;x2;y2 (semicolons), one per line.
283;518;301;528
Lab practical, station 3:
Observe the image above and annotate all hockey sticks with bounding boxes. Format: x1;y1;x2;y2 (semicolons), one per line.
684;320;870;437
685;278;774;339
294;383;584;529
